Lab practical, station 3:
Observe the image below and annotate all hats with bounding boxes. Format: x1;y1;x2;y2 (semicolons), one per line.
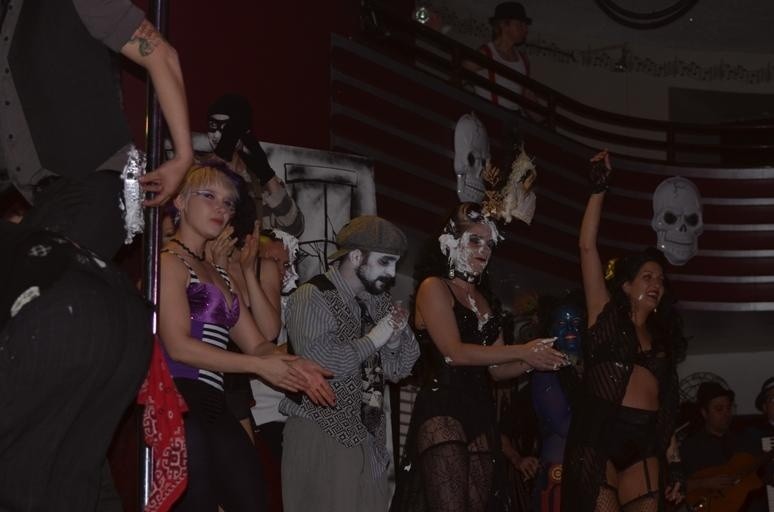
328;215;407;263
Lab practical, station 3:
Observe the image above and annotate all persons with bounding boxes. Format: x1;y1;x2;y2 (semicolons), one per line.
532;286;586;512
274;214;423;512
559;144;689;512
448;1;533;117
488;286;551;511
0;0;195;512
394;200;572;512
734;376;774;512
674;380;748;512
135;91;336;512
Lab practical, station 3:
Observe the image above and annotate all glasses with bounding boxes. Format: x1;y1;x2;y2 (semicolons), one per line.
187;188;236;212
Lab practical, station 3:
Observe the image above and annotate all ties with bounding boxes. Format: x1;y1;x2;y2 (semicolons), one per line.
355;295;384;433
